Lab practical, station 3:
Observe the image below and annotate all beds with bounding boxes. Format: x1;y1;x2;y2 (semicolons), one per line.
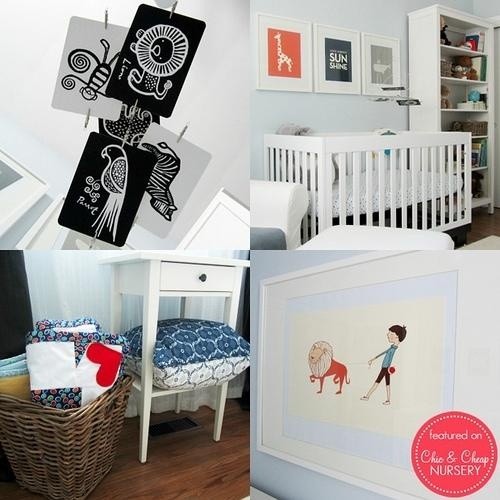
263;132;472;245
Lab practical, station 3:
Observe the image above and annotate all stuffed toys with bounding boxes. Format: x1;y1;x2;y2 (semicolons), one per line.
461;171;484;199
270;121;339;192
440;16;478;111
373;128;405;170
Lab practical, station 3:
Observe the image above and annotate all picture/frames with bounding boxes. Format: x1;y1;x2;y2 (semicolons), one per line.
256;250;500;500
313;24;361;94
361;32;401;97
257;14;312;92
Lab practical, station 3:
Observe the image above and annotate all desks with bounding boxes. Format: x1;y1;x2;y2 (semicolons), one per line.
99;252;250;464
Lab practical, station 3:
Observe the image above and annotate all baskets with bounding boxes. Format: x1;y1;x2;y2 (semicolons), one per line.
0;370;135;499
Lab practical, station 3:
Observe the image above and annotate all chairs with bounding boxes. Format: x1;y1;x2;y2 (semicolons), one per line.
250;180;309;250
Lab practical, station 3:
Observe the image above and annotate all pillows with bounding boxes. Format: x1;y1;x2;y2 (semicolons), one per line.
124;319;250;390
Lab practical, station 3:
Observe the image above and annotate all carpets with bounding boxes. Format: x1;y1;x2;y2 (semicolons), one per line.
454;235;499;250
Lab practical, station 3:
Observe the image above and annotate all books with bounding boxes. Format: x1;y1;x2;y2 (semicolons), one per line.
467;139;488;167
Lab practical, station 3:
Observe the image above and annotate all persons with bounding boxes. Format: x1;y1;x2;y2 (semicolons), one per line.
359;326;408;405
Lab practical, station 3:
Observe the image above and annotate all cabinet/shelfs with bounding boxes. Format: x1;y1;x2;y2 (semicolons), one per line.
407;4;494;215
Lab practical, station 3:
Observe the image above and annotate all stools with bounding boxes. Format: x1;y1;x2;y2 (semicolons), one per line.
296;226;454;250
